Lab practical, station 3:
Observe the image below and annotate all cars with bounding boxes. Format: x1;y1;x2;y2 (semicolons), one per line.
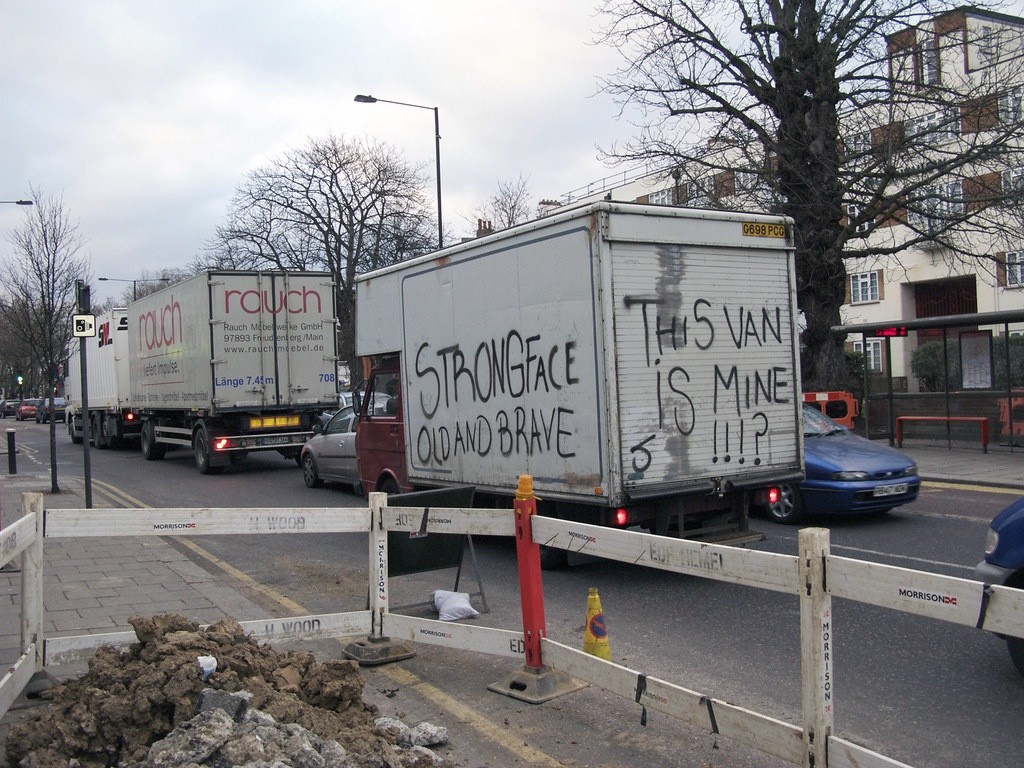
0;399;22;419
300;403;392;494
746;403;923;524
312;391;395;430
35;397;66;424
15;398;44;421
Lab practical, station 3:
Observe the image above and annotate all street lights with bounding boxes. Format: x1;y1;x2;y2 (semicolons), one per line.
97;278;172;302
353;94;445;249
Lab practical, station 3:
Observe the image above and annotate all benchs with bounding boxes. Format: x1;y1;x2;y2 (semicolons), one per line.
895;416;990;454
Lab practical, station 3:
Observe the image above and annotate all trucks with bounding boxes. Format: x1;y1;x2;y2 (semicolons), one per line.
126;267;341;475
347;201;806;571
62;306;149;449
